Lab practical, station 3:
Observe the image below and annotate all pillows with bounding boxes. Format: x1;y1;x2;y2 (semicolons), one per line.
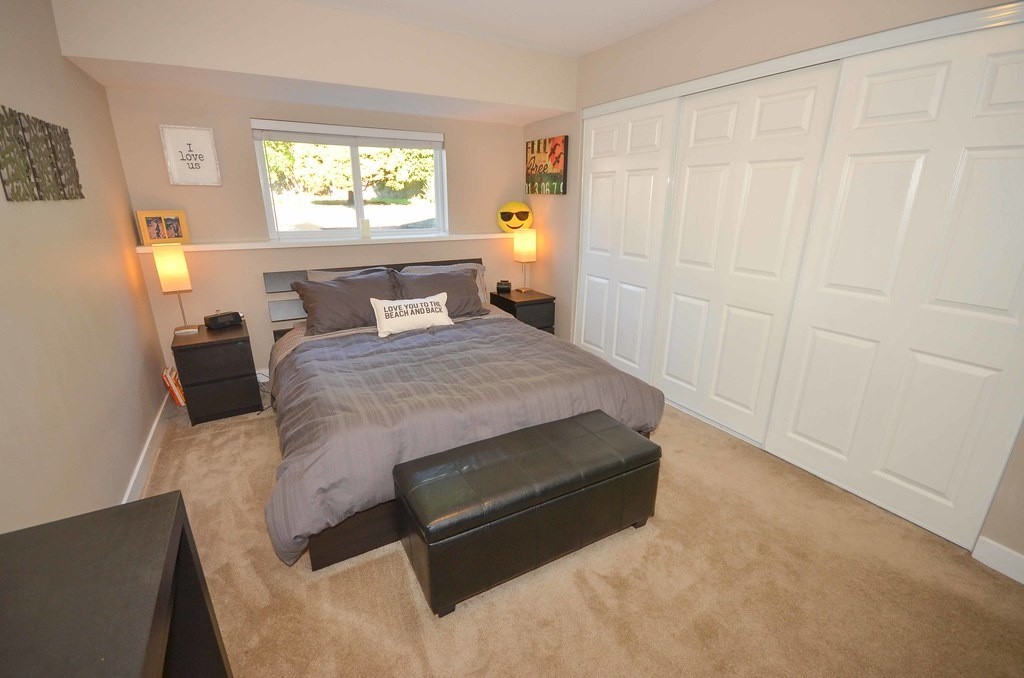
294;263;491;338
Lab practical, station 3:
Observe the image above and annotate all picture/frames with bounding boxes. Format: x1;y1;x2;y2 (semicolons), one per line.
159;125;222;187
136;210;193;246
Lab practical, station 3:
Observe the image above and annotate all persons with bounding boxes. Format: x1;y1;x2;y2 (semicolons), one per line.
155;222;161;239
169;221;180;237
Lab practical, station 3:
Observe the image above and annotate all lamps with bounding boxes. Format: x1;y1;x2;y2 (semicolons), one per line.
512;228;538;293
151;243;199;334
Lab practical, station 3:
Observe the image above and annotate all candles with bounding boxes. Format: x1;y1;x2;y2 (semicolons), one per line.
360;218;372;239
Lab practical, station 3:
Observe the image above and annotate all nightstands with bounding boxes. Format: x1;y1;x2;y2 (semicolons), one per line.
171;320;263;426
490;288;556;336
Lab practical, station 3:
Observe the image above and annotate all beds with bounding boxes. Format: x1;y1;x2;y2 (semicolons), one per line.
264;257;666;574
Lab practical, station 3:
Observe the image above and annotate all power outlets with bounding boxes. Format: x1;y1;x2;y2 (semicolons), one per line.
255;369;270;382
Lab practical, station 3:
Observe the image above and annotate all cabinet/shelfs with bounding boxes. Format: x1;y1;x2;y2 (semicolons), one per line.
570;0;1024;573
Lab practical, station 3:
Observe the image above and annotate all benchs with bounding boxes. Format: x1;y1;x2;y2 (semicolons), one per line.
390;410;663;618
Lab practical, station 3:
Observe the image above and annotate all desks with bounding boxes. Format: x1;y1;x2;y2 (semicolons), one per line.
0;490;235;678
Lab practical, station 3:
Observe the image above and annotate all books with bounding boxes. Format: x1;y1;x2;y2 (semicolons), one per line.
162;367;186;407
